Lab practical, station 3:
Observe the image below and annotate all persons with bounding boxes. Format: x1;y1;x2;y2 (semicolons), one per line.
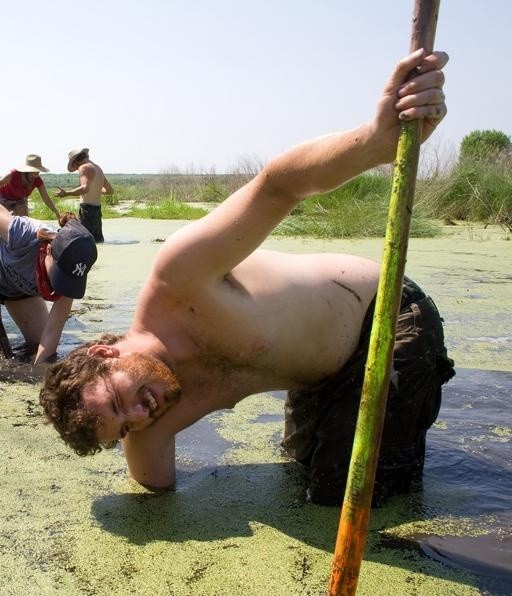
52;147;114;244
38;44;457;508
0;153;61;223
1;201;98;369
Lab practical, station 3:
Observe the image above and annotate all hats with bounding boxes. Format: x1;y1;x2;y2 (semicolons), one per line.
50;218;98;300
15;153;50;174
67;148;90;173
